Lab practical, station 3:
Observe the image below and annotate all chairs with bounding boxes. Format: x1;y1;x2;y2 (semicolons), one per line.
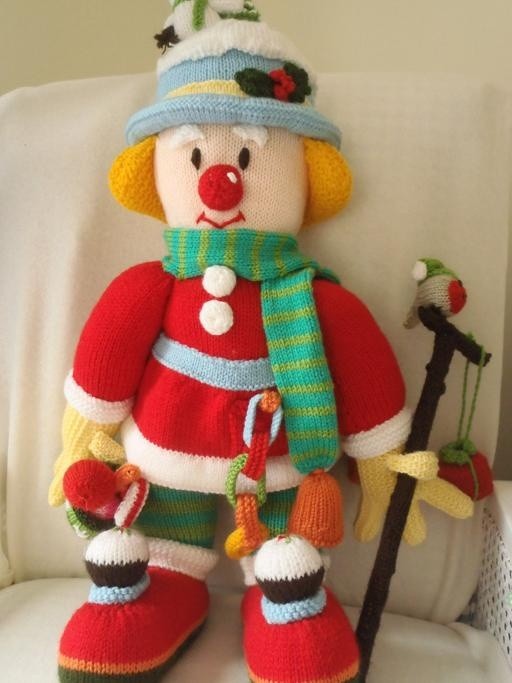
1;61;511;683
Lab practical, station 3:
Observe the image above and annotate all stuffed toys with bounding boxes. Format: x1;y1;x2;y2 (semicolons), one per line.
49;0;473;683
